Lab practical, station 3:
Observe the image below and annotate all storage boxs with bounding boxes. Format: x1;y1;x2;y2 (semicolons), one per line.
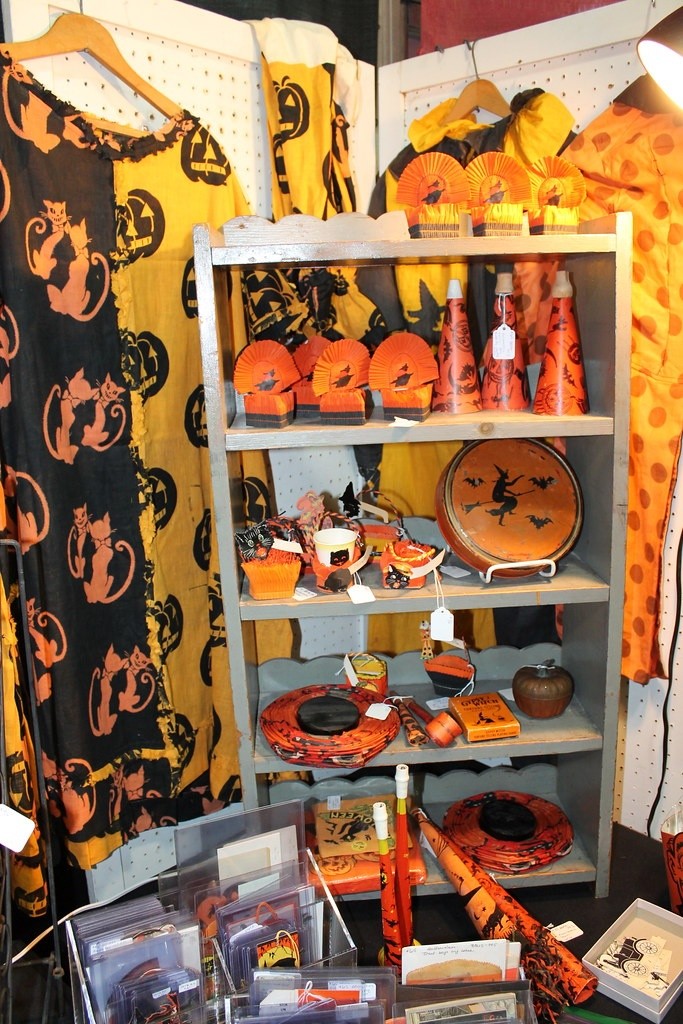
581;897;683;1024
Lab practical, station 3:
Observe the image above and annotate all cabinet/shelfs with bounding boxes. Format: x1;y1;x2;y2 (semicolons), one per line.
193;211;634;912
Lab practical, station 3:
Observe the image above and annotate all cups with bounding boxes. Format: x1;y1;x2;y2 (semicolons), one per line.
313;528;357;567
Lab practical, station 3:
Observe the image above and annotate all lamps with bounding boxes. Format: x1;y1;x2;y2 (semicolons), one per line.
635;8;683;112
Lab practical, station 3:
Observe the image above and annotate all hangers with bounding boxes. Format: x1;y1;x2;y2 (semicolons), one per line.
440;34;511;125
0;0;181;121
